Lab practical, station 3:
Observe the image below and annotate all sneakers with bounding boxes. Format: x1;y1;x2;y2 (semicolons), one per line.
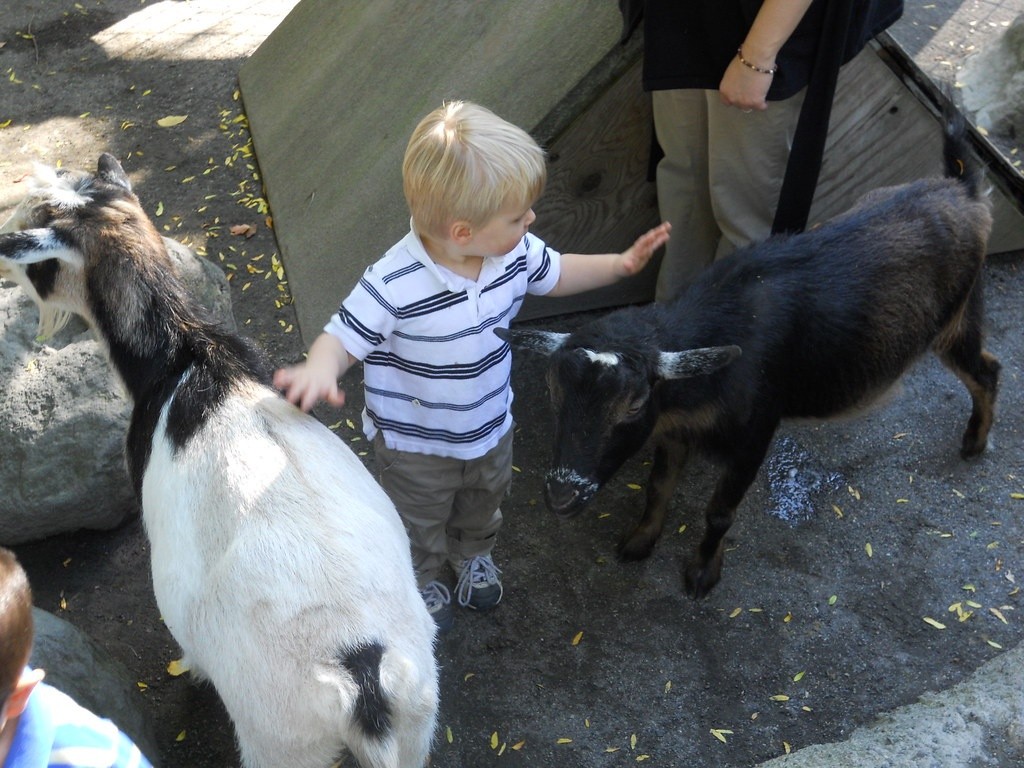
450;553;503;611
418;582;454;635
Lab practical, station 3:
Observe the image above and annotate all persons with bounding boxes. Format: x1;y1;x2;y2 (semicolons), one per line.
272;101;671;636
618;0;904;302
0;546;153;768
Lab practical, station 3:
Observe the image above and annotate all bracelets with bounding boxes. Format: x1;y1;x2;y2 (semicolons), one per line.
738;44;777;73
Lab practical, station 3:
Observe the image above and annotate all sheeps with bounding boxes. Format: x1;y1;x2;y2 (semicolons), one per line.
492;71;1002;602
0;152;442;768
